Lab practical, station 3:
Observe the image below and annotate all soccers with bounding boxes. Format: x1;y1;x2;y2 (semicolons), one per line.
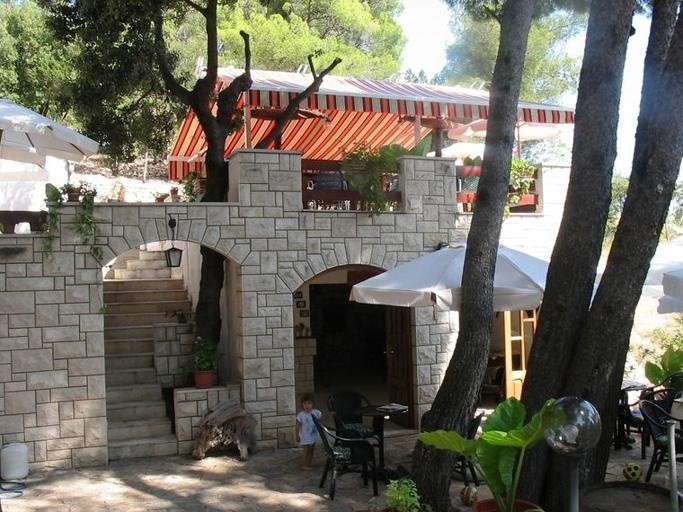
622;463;643;483
460;485;479;505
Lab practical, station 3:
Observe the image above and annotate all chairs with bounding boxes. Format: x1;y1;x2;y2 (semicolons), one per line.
326;392;377;478
478;366;505;406
616;388;677;459
639;400;683;482
641;373;683;399
311;413;378;500
453;412;484;486
620;385;648;406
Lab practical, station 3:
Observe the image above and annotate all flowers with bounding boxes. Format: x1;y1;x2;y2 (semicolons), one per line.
192;337;218;371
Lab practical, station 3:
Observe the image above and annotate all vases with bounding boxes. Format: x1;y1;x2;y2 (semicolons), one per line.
195;371;217;389
579;481;683;512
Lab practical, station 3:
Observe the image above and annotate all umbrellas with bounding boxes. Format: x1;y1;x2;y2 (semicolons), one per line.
642;237;683;284
0;97;99;166
349;237;603;313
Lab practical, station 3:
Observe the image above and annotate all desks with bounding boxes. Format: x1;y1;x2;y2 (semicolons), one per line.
487;351;520;369
343;401;408;481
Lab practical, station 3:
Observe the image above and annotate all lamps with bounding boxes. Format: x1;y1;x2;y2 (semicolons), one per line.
164;240;183;267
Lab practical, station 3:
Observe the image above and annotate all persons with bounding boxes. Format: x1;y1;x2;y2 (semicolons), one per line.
294;394;336;471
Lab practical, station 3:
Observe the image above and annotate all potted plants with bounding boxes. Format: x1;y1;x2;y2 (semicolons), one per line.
415;397;567;511
182;172;207;202
462;168;538;212
59;182;88;202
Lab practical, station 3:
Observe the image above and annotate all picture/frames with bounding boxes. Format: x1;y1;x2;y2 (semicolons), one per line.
294;291;310;317
